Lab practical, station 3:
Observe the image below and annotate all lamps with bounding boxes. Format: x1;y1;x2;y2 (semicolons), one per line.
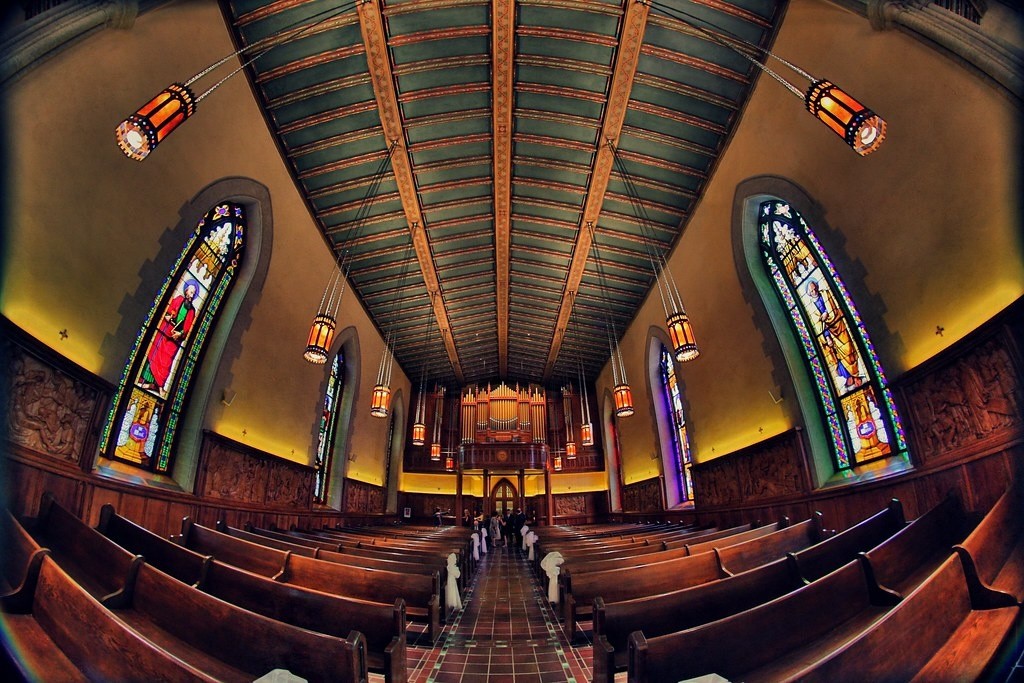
558;327;577;460
587;219;635;418
430;328;447;461
370;222;417;418
569;290;594;447
114;0;369;163
303;139;398;365
445;361;456;471
550;361;562;472
413;290;436;446
633;0;889;158
606;138;700;363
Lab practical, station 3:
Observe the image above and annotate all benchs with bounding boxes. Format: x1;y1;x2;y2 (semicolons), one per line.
522;475;1024;683
0;492;485;683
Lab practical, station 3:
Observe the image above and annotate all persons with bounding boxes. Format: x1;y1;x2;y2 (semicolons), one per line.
433;506;450;527
463;507;539;548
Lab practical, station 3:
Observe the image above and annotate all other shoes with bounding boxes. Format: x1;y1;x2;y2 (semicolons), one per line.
491;544;497;548
502;545;507;548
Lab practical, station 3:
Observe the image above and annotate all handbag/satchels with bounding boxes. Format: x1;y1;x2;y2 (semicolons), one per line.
489;529;495;537
